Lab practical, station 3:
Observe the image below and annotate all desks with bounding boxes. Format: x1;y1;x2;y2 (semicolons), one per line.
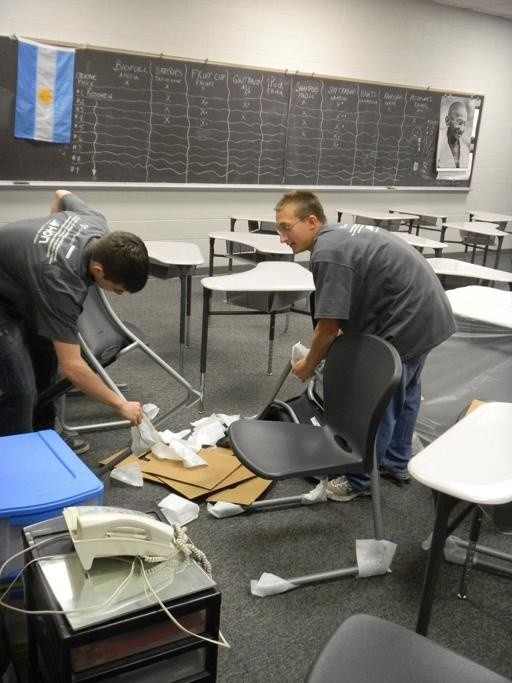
406;400;510;636
229;204;512;288
406;285;511;535
206;229;293;333
140;240;204;374
197;259;315;406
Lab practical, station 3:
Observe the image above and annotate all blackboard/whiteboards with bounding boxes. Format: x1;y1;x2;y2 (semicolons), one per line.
0;36;485;192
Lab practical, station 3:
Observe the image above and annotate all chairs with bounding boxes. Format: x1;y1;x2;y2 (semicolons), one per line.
214;334;400;596
43;218;196;444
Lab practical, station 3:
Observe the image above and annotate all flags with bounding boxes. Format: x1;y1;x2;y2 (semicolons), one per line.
14;33;75;144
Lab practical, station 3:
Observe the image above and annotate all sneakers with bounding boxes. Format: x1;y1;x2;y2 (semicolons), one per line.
326;476;372;502
59;431;90;455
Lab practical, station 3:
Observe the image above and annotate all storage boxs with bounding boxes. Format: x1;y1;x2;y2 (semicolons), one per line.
0;428;112;582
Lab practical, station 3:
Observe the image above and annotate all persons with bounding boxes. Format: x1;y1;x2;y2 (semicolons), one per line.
438;102;470;168
274;190;458;502
0;189;149;454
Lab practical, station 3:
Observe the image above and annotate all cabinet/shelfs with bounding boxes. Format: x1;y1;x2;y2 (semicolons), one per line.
24;541;219;683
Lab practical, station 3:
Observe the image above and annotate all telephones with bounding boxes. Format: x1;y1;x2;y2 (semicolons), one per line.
63;506;175;570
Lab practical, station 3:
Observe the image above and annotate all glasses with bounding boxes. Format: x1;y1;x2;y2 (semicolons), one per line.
277;215;309;235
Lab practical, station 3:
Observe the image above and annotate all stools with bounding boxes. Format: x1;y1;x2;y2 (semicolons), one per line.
306;609;512;680
